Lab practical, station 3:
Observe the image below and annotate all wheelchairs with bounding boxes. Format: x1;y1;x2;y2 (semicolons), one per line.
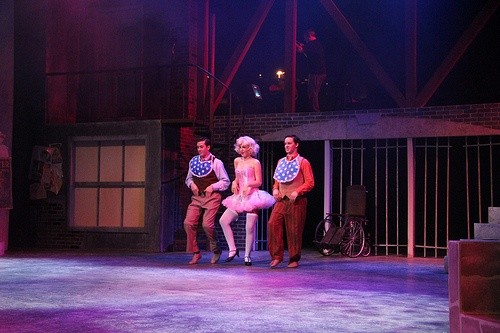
311;185;373;258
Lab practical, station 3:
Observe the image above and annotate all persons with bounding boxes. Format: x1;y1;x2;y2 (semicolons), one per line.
267;135;315;269
218;136;276;267
183;138;231;265
296;27;328;112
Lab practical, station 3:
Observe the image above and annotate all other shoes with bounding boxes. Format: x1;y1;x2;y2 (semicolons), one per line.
210;249;222;264
287;260;300;268
269;259;282;267
189;252;202;265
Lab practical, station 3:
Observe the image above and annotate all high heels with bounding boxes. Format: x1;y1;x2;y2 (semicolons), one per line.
244;256;252;266
225;248;240;263
